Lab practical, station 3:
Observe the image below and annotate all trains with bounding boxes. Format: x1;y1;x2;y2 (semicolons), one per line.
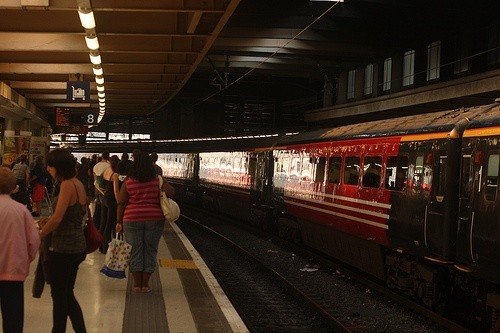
70;101;500;294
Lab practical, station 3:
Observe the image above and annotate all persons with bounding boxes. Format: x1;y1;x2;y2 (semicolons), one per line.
0;166;42;333
74;149;175;255
116;149;175;296
38;148;87;333
11;155;62;217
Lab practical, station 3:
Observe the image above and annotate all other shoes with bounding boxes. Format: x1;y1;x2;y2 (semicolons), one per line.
141;288;149;293
133;287;140;293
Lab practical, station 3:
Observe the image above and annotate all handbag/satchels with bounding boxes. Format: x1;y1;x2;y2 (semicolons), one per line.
84;198;104;255
97;176;105;190
158;175;181;223
99;230;133;279
102;172;123;209
